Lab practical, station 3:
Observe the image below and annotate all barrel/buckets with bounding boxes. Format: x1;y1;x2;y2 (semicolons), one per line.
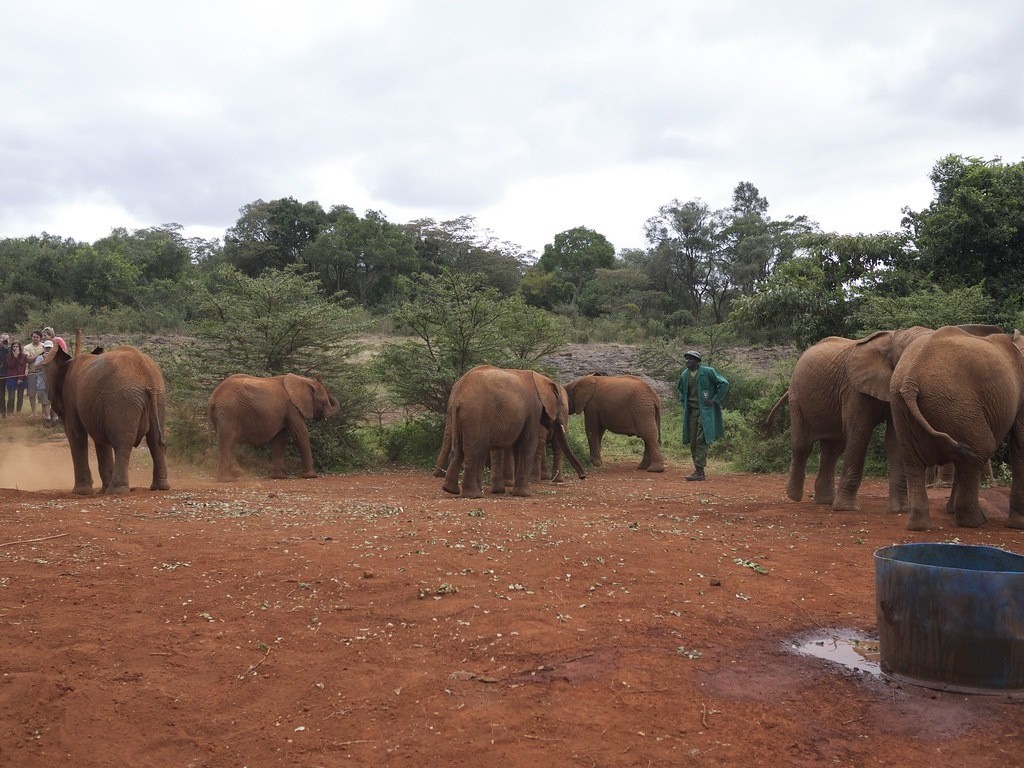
875;544;1024;697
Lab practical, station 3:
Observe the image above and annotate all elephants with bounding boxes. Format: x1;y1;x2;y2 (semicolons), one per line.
562;372;666;472
432;364;586;500
39;330;171;498
206;371;340;482
766;325;1024;531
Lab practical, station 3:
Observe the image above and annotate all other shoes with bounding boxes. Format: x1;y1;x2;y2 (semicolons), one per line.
0;414;6;418
685;467;705;481
45;416;50;421
53;416;59;422
8;412;15;417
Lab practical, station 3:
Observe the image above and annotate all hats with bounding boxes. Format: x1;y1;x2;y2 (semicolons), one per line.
44;340;54;348
684;351;702;361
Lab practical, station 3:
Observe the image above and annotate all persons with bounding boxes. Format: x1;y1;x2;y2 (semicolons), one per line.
679;351;730;480
0;331;9;418
23;331;44;420
6;340;28;418
34;340;59;428
41;327;67;353
